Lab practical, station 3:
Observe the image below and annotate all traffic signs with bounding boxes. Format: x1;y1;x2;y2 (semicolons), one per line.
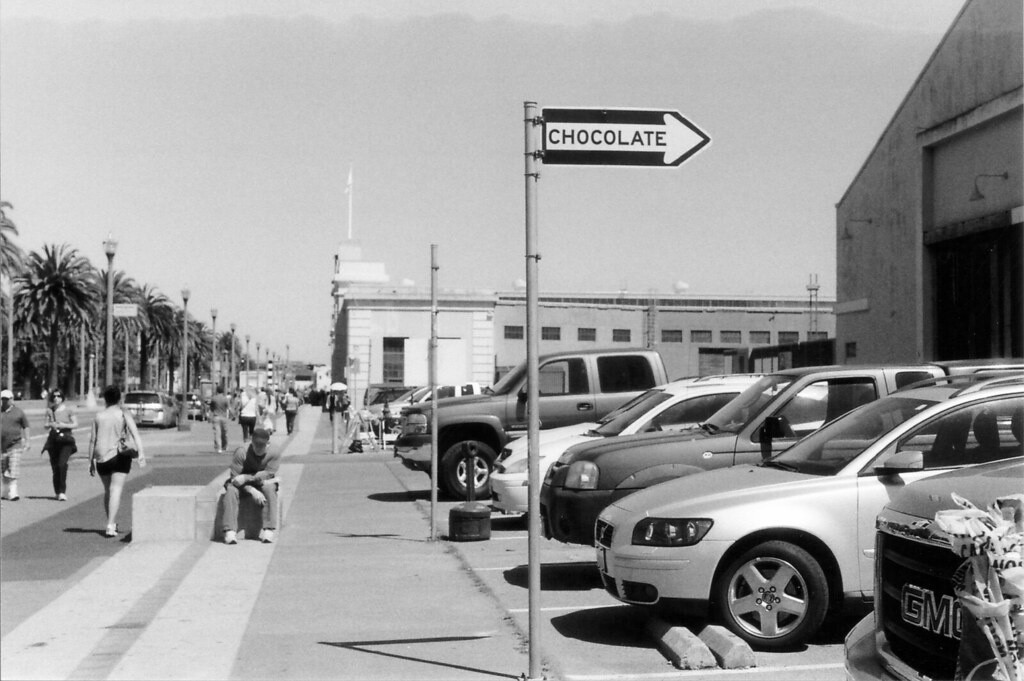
542;107;712;168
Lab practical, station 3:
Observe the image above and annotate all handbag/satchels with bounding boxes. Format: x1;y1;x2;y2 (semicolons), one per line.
253;412;274;434
118;411;139;458
55;432;74;445
239;416;241;424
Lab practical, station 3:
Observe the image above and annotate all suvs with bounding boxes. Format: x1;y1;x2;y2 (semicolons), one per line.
587;359;1018;648
538;344;1022;547
175;391;207;421
396;346;668;498
121;389;181;426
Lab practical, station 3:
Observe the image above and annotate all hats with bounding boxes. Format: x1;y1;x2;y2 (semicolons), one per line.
252;428;269;443
1;390;14;399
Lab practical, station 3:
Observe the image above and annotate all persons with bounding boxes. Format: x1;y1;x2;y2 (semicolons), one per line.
208;385;350;454
88;385;147;537
41;390;79;501
0;390;31;501
221;429;281;544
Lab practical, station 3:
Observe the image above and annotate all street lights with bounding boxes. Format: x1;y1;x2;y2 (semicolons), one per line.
178;289;193;431
210;308;218;383
230;322;237;391
245;335;250;373
99;231;119;399
264;345;291;374
256;344;260;370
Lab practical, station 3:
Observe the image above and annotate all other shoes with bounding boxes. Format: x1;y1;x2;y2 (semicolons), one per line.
10;496;20;501
215;448;227;453
55;494;66;501
1;497;9;500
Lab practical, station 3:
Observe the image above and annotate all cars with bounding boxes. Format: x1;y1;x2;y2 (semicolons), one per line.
492;378;708;472
362;387;437;433
494;374;830;515
841;453;1024;681
361;382;481;436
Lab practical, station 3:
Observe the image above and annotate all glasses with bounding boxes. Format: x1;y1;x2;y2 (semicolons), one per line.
52;394;61;399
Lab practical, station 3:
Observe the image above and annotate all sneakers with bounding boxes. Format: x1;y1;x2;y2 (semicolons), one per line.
106;523;118;536
263;529;274;543
225;530;237;544
